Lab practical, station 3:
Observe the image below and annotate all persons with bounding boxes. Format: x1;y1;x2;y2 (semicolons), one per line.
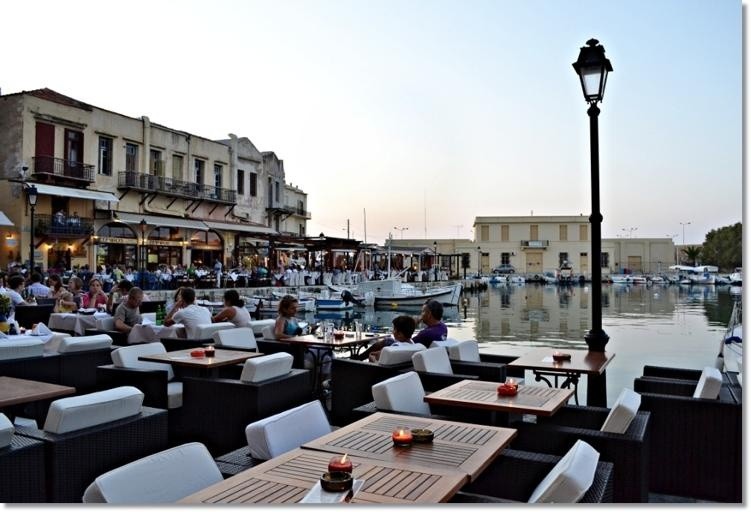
70;212;80;227
54;209;66;227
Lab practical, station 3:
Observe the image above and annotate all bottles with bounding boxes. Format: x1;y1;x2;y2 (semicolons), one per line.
156;304;167;325
22;288;35;302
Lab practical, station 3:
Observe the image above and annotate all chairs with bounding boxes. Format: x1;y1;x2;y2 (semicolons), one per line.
1;261;451;307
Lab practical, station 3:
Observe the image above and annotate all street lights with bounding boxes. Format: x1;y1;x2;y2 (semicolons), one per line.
570;36;613;407
680;221;691;244
622;226;638;239
616;235;621;238
391;225;409;239
432;241;440;280
342;228;354;238
319;232;324;284
666;234;679;241
140;218;148;287
477;245;480;277
25;183;41;279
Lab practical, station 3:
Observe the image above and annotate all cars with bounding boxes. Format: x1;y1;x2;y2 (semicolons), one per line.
492;263;516;274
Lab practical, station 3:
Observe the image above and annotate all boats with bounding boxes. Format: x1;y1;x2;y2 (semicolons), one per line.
462;271;555;290
610;264;742;285
188;279;465;319
555;258;586;286
714;298;742;388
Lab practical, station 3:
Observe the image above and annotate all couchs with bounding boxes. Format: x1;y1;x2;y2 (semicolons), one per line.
0;307;742;504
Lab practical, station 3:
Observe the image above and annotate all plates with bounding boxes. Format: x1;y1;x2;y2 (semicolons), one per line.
320;472;353;492
553;354;572;361
333;333;345;338
78;311;96;315
497;385;517;395
411;428;435;441
191;350;205;358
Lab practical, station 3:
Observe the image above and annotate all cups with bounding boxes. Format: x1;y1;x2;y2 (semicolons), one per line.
20;328;25;334
328;456;353;473
205;347;216;356
71;306;78;313
392;425;413;446
31;324;38;335
506;376;519;390
321;318;335;340
355;319;362;337
59;299;64;313
